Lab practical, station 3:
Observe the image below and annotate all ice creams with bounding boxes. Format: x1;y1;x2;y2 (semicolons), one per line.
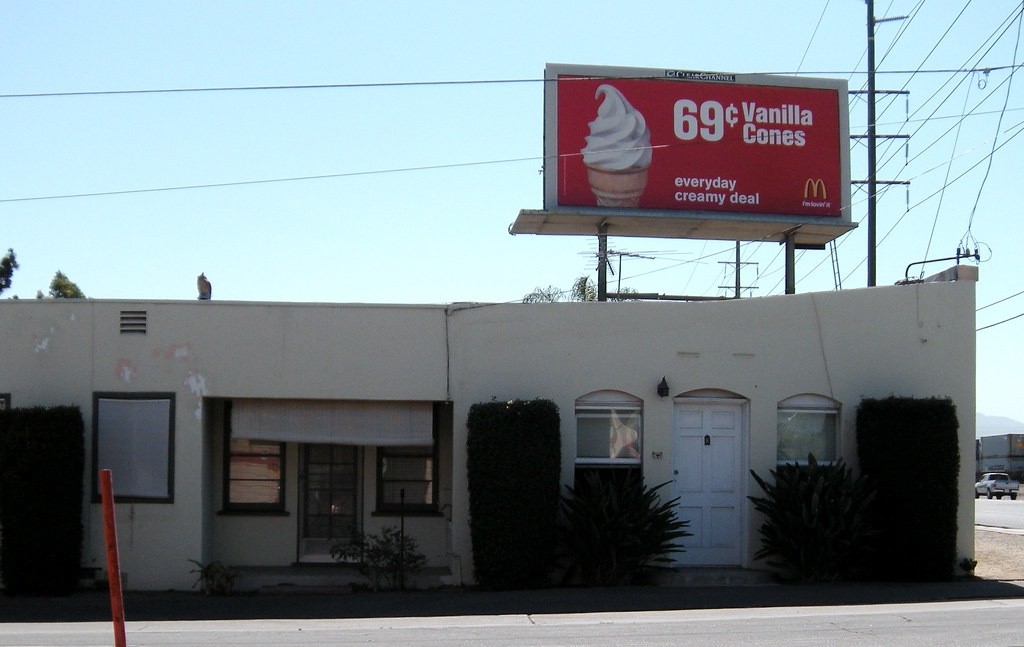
581;83;652;208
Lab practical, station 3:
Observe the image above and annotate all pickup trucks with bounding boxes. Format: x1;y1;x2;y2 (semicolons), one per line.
975;472;1020;499
